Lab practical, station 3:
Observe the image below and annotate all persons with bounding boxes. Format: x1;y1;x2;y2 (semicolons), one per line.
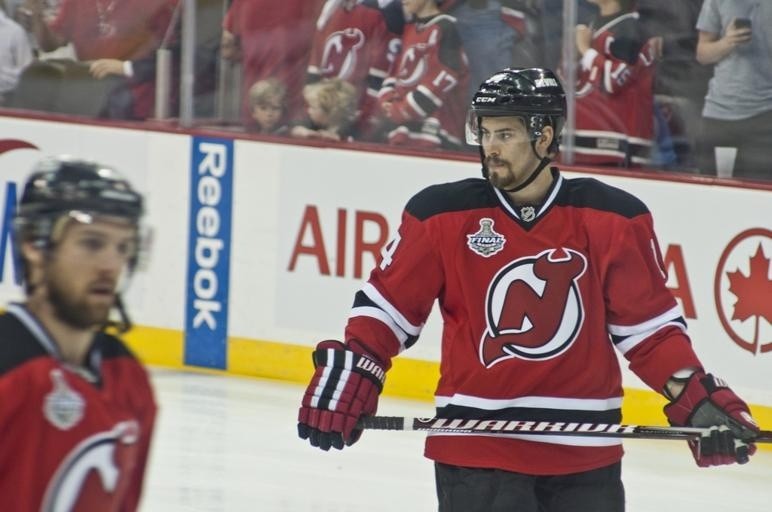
296;66;760;512
1;161;155;512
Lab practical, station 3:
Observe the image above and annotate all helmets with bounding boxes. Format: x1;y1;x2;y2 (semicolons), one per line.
464;66;566;148
11;155;145;332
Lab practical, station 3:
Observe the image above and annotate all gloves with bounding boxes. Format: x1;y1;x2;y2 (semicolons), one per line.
663;368;759;466
297;339;386;451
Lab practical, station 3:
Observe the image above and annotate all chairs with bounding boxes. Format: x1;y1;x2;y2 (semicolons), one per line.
10;60;124;120
447;1;538;110
